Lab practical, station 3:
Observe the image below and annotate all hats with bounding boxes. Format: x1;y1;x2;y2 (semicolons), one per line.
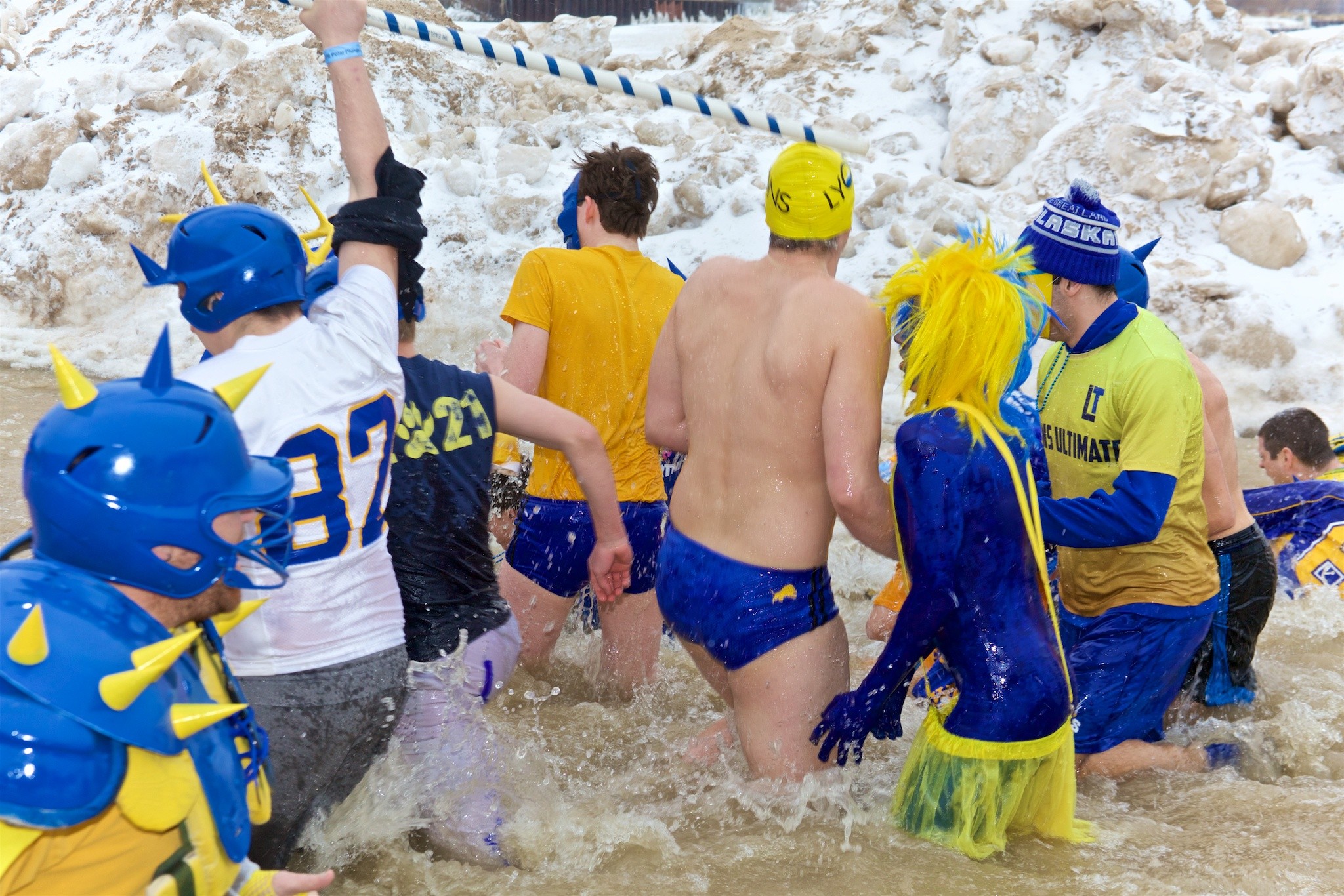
764;144;854;242
1012;181;1121;285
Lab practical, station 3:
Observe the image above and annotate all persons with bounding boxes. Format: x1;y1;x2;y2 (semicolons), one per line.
644;142;900;784
863;177;1247;779
381;314;635;858
1113;236;1279;707
810;219;1106;862
1242;407;1344;603
2;321;335;896
472;143;688;692
130;0;426;871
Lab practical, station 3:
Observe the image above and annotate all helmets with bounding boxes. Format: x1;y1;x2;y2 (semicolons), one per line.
34;329;293;592
131;204;306;323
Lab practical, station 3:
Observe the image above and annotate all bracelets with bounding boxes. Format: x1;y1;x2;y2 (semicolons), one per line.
323;41;362;65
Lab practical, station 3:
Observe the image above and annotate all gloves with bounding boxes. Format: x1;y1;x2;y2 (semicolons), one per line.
810;689;877;767
